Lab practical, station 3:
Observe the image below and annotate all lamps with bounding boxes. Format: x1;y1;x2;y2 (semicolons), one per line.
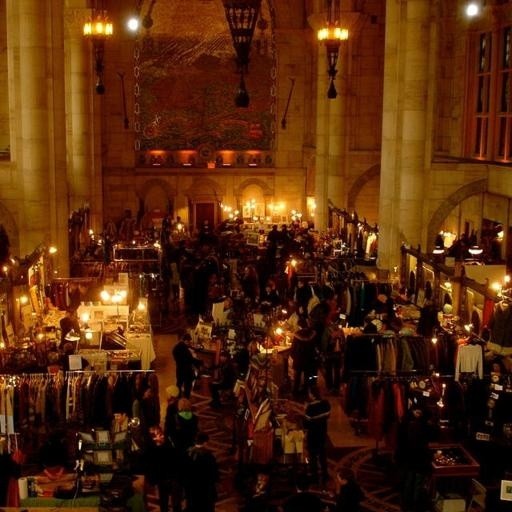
83;0;113;93
316;0;349;97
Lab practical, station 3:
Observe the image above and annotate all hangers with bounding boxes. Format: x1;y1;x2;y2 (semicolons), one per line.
453;330;485;350
346;333;449;354
0;369;157;392
297;279;393;295
352;369;473;398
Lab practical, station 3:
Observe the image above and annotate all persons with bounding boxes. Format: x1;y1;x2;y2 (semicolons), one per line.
0;216;512;512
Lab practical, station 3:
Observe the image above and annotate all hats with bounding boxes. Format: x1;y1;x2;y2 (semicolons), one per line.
166;386;179;397
178;399;191;410
195;432;208;444
